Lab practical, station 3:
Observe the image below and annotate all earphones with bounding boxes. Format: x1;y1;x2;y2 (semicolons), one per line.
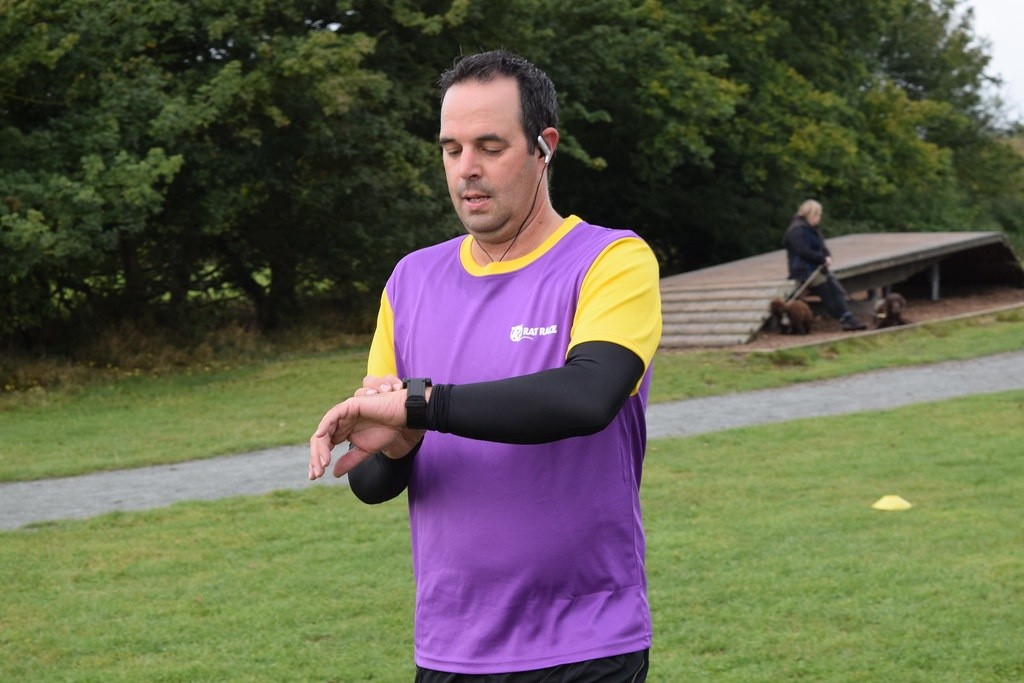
537;135;552;164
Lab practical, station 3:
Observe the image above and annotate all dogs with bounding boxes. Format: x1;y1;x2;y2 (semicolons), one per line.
771;300;812;335
872;292;915;330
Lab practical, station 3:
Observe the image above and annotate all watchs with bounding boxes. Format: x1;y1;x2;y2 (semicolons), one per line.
400;377;433;429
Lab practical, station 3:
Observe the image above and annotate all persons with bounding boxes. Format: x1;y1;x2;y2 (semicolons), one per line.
306;49;664;683
783;199;868;330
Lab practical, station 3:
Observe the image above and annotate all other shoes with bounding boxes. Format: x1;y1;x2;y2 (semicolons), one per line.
842;316;867;330
